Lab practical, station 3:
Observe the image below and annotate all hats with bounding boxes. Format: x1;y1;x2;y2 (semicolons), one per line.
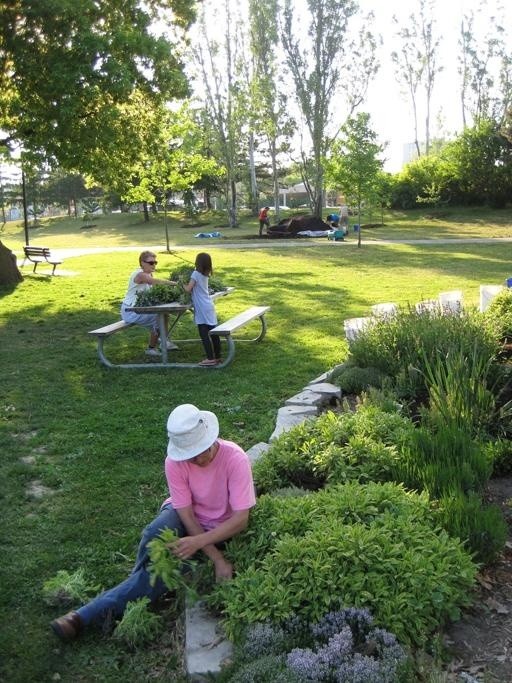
167;403;220;462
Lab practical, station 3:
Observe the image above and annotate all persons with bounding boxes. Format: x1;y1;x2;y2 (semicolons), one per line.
121;251;180;356
183;253;222;366
327;214;340;228
50;403;257;645
259;207;271;236
340;204;349;234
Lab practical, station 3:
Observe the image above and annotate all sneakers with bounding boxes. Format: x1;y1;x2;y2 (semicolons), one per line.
52;612;83;641
199;359;217;365
160;340;179;351
145;348;160;355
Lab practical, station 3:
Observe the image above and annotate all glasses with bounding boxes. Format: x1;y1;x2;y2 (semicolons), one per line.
144;261;159;266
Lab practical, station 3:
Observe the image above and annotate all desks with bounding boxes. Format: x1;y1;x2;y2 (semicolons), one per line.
125;287;235;369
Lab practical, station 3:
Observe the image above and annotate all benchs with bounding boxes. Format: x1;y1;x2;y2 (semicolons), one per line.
208;306;271;369
23;246;62;276
89;319;136;368
